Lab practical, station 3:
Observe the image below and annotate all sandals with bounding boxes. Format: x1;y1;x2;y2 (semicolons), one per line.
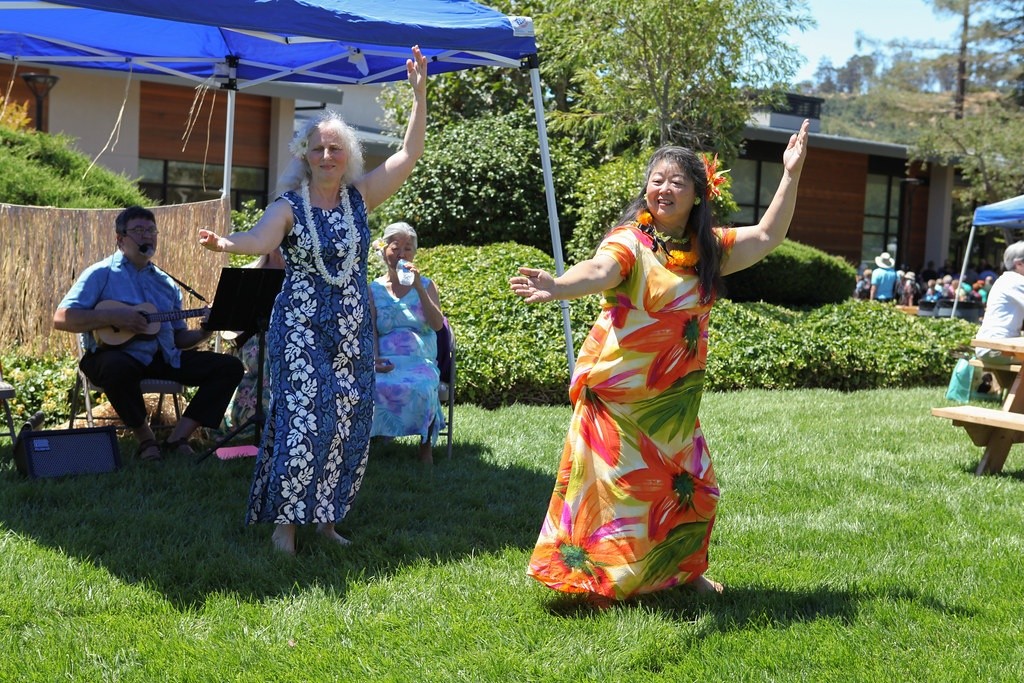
136;439;161;461
162;437;198;456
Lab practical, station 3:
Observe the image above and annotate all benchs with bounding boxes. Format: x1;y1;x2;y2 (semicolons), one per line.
930;406;1024;432
969;359;1021;373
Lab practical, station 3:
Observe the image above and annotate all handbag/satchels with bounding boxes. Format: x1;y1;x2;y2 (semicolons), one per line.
946;356;1008;403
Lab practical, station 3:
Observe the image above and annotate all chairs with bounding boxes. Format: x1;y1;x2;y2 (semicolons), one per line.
68;332;183;433
435;317;455;461
0;373;17;448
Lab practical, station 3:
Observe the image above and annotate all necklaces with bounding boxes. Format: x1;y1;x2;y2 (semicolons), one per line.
301;176;357;287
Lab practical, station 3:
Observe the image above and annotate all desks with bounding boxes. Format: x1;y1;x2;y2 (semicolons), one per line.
968;334;1024;474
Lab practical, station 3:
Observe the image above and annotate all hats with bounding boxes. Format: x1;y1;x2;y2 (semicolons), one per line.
875;252;895;268
904;271;916;280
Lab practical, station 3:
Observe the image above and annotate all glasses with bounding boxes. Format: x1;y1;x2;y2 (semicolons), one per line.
124;227;159;235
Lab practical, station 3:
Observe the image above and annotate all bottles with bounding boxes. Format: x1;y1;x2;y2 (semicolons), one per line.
395;259;415;286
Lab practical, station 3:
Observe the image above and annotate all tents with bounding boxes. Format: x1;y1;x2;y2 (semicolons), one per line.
951;194;1024;318
0;0;575;378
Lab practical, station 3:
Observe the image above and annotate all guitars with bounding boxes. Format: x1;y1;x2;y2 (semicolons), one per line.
92;299;212;351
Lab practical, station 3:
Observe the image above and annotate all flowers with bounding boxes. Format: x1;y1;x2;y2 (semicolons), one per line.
287;135;309;158
700;149;732;200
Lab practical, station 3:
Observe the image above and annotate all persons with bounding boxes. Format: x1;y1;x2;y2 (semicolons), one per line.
54;46;443;554
855;251;993;304
513;117;808;610
977;240;1024;393
